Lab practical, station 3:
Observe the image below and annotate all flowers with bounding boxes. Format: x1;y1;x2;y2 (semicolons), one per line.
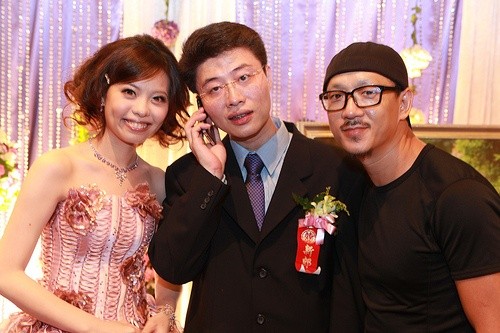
289;187;351;246
0;132;21;212
154;19;179;48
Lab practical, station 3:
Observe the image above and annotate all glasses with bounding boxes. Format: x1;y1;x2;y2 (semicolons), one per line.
319;84;395;111
198;65;268;104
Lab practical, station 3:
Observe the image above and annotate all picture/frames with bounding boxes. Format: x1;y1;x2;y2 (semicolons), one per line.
296;121;500;192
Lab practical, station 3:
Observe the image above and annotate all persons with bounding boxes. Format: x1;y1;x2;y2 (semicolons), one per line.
85;138;140;187
317;41;500;333
1;34;183;332
148;21;367;332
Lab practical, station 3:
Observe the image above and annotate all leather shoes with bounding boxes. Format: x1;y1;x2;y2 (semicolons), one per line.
243;152;265;231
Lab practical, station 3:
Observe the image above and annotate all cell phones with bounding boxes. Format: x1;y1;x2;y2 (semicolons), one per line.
196;95;216;146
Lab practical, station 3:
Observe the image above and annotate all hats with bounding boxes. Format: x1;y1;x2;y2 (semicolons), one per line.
323;41;408;92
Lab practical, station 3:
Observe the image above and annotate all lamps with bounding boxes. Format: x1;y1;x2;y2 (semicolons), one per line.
400;45;432;124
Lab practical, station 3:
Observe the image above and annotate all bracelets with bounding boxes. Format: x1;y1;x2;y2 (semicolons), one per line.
153;304;179;330
